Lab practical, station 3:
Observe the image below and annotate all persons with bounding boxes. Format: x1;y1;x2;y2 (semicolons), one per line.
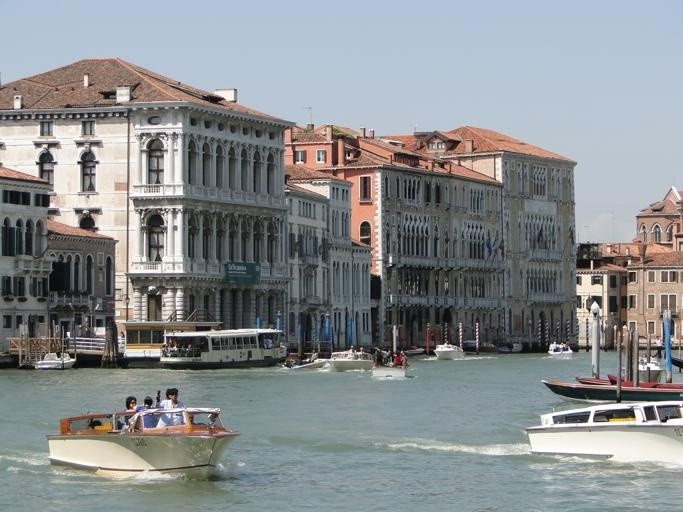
264;336;272;349
125;388;186;428
656;336;661;358
375;349;406;367
162;338;207;357
552;342;568;352
347;346;364;360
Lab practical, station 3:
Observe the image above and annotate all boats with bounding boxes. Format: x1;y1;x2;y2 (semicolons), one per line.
36;352;77;370
160;328;286;369
526;355;683;458
45;408;242;480
548;344;573;355
433;345;464;360
284;352;405;382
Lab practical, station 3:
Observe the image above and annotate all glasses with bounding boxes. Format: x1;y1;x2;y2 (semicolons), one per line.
131;402;136;404
